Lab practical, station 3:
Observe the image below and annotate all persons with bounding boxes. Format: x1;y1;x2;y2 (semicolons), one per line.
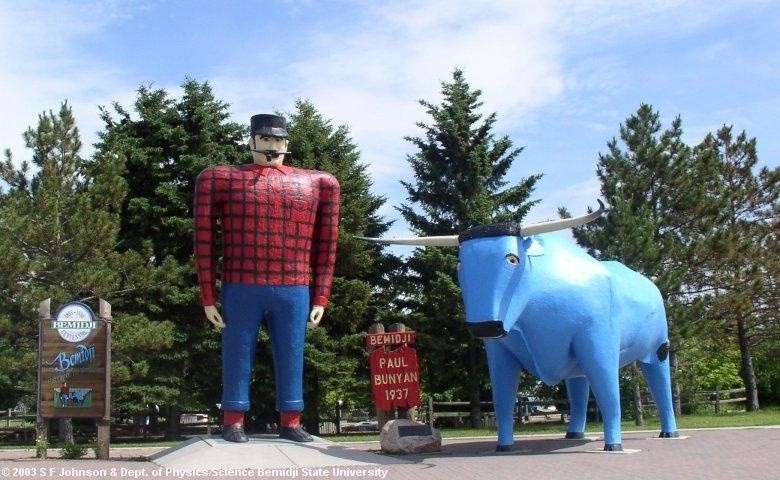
192;112;341;443
60;382;70;408
